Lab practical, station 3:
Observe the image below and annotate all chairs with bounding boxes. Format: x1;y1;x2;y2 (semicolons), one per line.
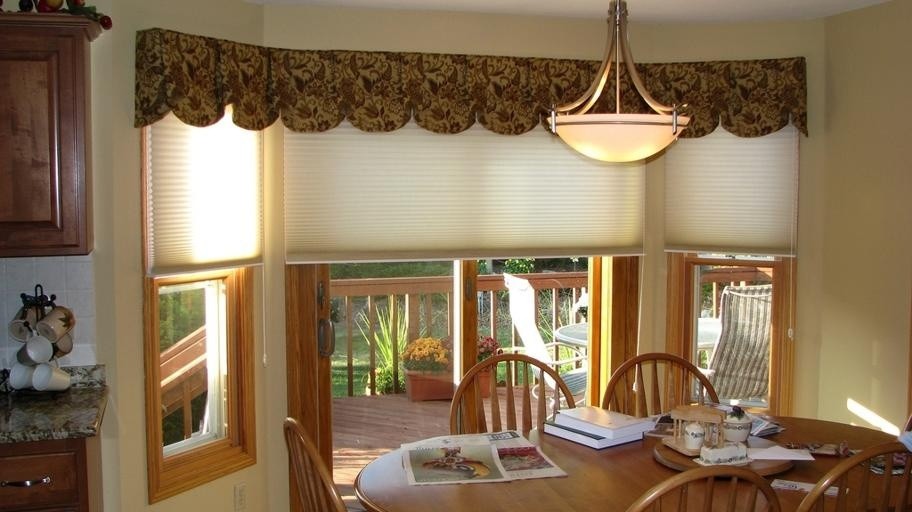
449;353;576;436
283;416;347;511
627;465;782;512
602;352;719;419
694;284;771;407
798;442;912;512
503;272;587;422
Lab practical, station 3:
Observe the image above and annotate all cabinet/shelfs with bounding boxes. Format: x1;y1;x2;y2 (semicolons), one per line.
0;11;103;259
0;387;110;512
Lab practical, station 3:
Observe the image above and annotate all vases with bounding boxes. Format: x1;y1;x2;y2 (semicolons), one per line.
407;371;491;403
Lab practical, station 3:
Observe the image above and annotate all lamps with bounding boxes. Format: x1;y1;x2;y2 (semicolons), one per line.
546;0;691;163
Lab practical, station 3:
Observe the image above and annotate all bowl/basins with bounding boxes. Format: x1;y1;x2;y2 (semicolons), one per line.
723;420;752;442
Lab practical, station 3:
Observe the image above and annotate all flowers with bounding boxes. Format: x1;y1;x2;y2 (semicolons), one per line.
402;335;498;371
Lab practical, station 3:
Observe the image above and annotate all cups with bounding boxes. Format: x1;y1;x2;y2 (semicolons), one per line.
8;305;36;341
17;336;53;366
685;426;705;451
33;363;70;390
56;331;73;357
9;362;31;388
36;306;75;343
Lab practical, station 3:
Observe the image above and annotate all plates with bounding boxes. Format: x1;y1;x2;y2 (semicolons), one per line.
695;455;752;466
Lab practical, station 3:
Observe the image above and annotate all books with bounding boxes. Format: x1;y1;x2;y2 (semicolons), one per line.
544;420;644;449
555;405;653;441
